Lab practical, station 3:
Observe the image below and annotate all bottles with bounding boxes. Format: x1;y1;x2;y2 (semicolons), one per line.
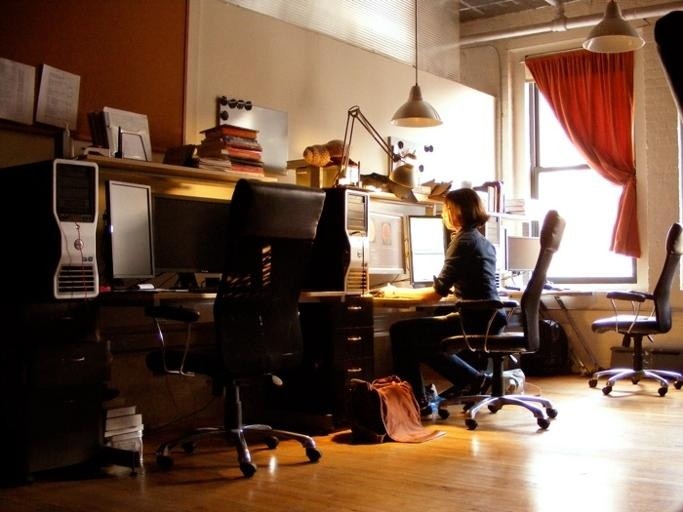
70;139;74;157
62;123;70;158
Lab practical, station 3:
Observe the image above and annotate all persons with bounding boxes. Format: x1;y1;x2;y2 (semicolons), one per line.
375;188;507;420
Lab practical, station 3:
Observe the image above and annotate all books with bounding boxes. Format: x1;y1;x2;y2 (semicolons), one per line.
101;397;144;463
88;106;152;161
195;123;265;177
487;184;526;214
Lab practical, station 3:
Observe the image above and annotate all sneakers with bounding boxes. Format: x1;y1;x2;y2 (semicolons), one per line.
419;401;432;420
462;372;492;411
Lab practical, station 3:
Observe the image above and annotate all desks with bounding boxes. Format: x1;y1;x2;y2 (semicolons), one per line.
329;185;592;412
502;212;611;378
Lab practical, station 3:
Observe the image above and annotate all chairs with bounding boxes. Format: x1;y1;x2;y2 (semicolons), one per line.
588;223;683;398
437;210;566;431
180;176;327;478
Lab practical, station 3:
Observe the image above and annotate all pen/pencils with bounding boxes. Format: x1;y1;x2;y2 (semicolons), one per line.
391;274;400;284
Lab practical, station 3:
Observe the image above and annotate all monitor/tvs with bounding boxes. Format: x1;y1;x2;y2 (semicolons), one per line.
107;179;157;279
367;212;409;290
506;236;541;291
405;215;449;285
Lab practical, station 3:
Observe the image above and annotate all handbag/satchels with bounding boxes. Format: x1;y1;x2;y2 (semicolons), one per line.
486;368;525;396
344;374;447;444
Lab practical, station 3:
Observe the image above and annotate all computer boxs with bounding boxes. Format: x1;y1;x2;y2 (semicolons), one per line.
7;158;100;302
320;186;370;296
479;223;501;293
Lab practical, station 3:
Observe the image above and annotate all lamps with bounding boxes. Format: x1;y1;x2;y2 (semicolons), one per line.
387;1;442;128
331;106;424;191
583;1;646;55
